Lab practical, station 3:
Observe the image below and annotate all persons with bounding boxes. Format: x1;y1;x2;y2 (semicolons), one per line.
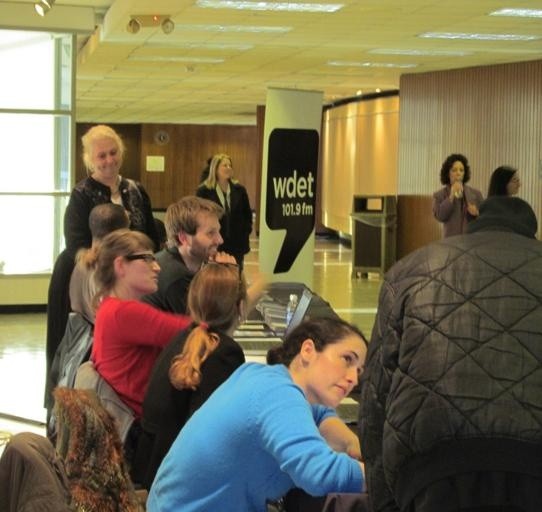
63;124;160;259
120;262;249;488
196;153;252;272
140;195;237;314
357;195;542;512
68;202;132;323
75;226;270;414
430;153;485;239
144;314;365;511
200;158;212;182
487;165;522;197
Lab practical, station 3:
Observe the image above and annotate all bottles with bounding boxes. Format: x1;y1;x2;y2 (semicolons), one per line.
455;180;463;199
286;293;300;326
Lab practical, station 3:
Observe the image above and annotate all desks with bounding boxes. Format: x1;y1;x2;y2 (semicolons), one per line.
234;282;360;431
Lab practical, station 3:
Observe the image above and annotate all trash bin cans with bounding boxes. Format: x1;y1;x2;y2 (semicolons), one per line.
352;193;397;279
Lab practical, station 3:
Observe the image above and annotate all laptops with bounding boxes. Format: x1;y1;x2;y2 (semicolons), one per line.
232;287;314;356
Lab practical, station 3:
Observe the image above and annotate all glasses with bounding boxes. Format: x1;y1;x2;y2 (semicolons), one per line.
125;254;157;264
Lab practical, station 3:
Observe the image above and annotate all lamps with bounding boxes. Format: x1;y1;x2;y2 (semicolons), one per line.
34;0;54;17
128;15;173;33
